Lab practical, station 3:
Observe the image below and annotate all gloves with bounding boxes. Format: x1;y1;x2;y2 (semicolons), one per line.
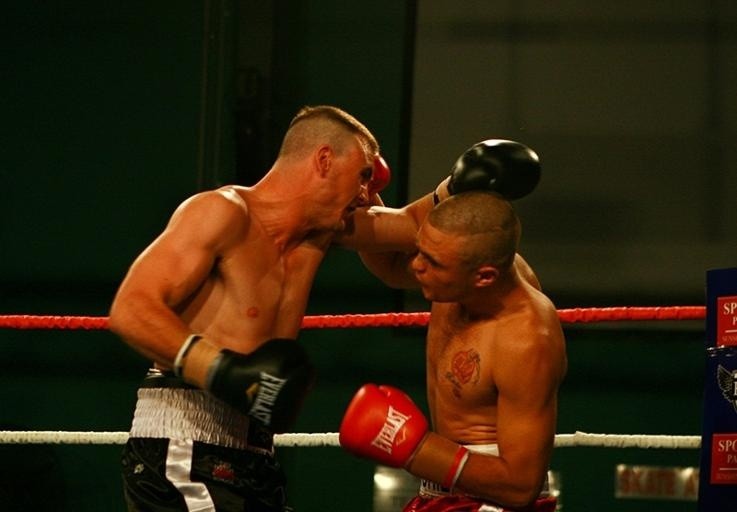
448;139;540;200
365;152;390;194
339;384;471;490
172;335;314;433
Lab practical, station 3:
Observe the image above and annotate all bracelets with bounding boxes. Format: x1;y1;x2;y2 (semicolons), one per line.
443;446;471;493
165;332;203;379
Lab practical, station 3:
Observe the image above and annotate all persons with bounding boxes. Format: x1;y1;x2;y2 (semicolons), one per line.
105;103;541;511
346;152;570;511
